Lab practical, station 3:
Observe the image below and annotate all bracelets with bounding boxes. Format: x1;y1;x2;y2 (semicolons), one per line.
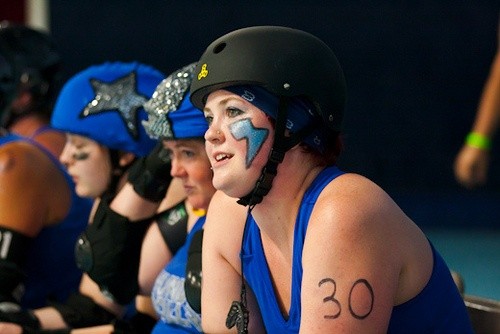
465;132;493;151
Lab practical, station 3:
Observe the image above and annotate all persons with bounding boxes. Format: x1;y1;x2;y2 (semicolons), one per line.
189;25;476;334
0;24;95;309
0;61;165;334
72;61;226;334
452;46;500;189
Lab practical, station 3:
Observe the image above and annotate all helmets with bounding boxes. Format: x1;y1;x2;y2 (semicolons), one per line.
190;26;346;135
0;23;75;99
51;61;166;155
141;60;209;141
